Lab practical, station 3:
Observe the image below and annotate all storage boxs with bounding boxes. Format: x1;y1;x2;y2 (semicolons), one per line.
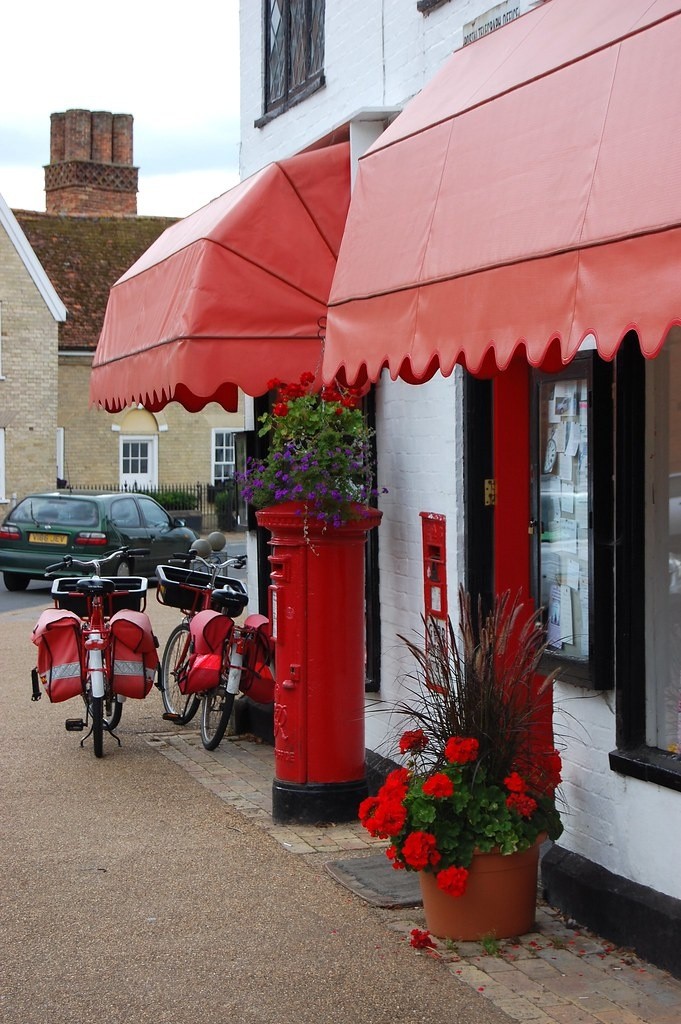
50;576;148;618
154;564;248;618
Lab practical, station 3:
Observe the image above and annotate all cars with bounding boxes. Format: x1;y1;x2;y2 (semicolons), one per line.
1;489;204;592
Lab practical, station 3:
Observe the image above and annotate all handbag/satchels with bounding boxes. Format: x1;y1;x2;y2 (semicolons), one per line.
243;612;279;702
30;607;86;704
106;609;158;698
176;608;235;696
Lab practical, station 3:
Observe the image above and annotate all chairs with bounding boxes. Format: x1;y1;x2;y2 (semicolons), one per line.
70;504;90;520
37;505;59;518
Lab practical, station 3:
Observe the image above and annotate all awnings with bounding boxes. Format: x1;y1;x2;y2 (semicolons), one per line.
314;0;681;393
92;138;351;413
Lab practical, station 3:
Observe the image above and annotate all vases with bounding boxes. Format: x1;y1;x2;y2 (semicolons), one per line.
419;833;548;942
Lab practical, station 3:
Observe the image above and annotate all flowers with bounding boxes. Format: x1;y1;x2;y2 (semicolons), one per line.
410;928;440;956
232;371;388;556
357;581;607;900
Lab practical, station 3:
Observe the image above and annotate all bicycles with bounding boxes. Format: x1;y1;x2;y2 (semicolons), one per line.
154;551;274;752
42;543;153;760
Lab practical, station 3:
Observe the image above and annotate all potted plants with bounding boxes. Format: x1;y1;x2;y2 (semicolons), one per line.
213;490;235;531
